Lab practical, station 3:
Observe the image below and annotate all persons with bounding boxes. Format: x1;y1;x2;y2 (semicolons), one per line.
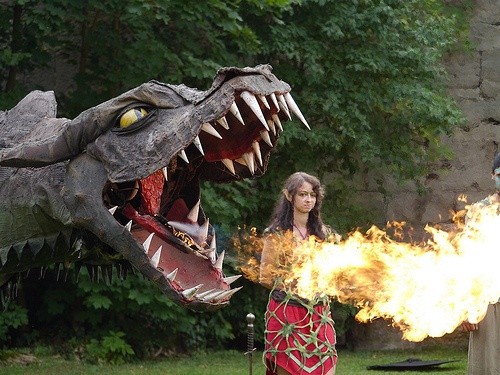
259;171;339;375
